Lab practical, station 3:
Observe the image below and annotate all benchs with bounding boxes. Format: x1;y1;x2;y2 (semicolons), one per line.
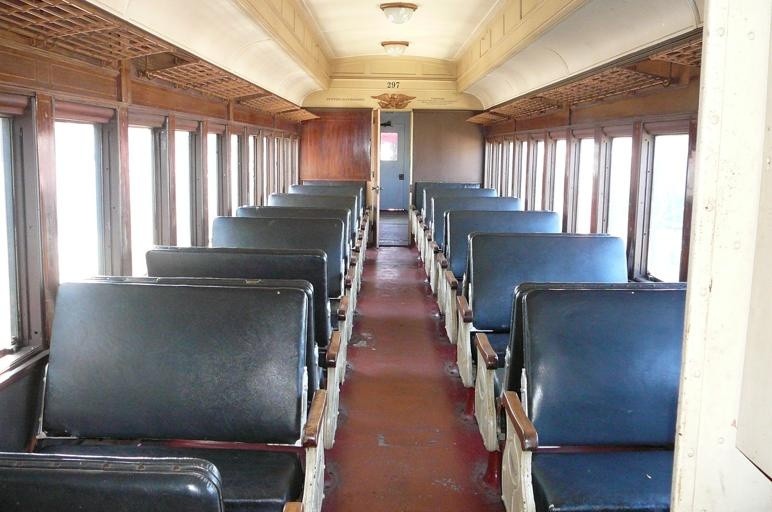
143;179;379;386
497;287;689;510
32;270;333;505
407;179;685;453
2;444;324;512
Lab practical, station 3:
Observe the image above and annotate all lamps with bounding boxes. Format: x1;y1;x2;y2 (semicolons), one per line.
380;3;417;24
381;41;408;57
